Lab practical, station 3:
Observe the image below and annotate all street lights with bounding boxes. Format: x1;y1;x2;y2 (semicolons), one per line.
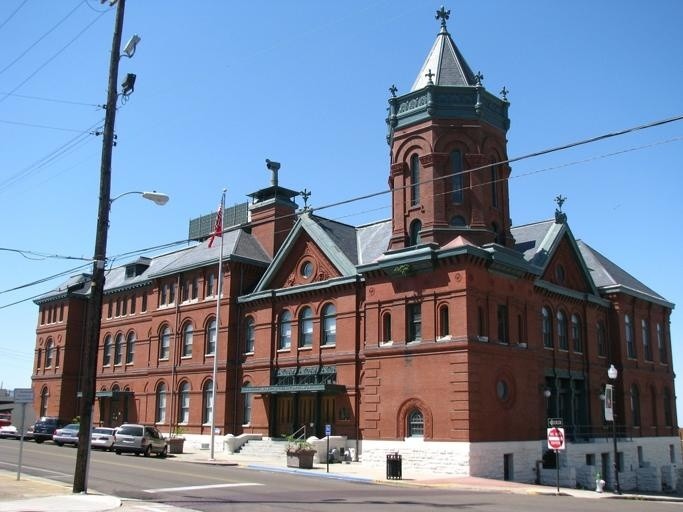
73;190;171;495
607;362;622;497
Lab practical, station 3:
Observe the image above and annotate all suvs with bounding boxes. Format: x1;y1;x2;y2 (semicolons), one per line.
113;423;168;458
32;416;60;443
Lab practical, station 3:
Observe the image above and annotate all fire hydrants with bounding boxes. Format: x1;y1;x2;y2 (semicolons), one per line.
595;472;605;493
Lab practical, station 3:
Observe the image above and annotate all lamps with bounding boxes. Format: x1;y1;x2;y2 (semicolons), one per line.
598;383;607;400
539;382;553;398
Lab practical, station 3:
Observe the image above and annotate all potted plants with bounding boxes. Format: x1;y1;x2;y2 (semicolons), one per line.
286;437;316;468
165;428;186;453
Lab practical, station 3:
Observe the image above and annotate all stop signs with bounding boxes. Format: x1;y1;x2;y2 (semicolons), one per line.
547;427;565;450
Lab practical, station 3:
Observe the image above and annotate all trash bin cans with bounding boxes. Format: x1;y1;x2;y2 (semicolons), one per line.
387;452;402;480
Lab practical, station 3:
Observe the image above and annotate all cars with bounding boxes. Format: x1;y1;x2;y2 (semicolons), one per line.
52;423;80;446
0;425;35;440
91;426;117;452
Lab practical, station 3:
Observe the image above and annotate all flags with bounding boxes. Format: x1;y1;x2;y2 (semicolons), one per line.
207;191;223;247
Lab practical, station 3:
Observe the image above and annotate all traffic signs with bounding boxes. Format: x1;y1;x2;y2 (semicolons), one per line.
547;417;564;428
